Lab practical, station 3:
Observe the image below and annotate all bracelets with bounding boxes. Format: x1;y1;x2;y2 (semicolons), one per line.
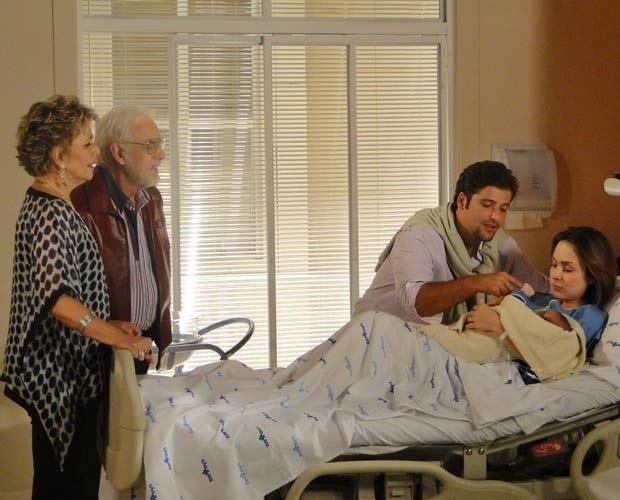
498;329;508;341
78;311;96;333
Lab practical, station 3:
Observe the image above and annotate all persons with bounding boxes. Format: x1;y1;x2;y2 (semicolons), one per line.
72;106;172;491
352;161;556;327
465;224;618;383
543;309;572;331
1;95;153;500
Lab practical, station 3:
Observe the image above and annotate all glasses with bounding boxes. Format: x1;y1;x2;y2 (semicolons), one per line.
118;138;165;154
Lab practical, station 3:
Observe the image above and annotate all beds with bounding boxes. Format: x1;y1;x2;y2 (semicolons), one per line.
101;276;620;500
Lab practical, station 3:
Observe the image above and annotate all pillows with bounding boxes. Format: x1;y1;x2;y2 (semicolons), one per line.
585;287;620;370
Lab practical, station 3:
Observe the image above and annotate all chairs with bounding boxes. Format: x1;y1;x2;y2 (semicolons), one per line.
151;315;255;362
